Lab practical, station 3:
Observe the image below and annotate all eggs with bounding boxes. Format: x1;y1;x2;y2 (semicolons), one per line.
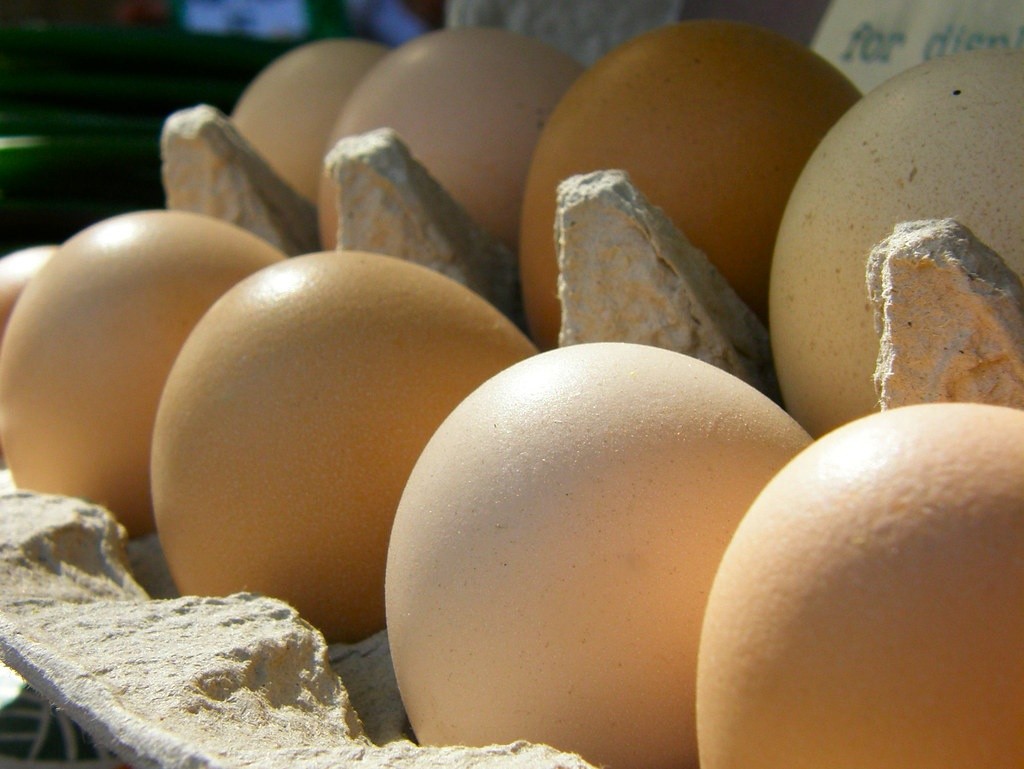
0;22;1024;769
